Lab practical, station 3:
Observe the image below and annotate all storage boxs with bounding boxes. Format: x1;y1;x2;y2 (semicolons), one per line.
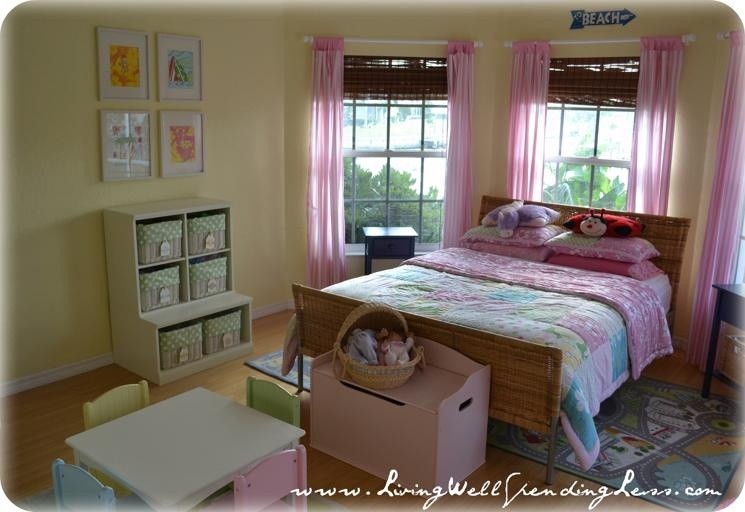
133;218;183;269
200;309;242;356
157;322;202;369
138;265;181;313
187;212;226;259
186;257;229;302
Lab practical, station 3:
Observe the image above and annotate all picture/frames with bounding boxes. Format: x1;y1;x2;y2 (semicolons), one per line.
97;108;154;184
153;32;205;103
157;108;206;180
94;24;151;103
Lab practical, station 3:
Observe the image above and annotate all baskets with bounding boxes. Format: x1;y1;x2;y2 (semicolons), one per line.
331;302;425;389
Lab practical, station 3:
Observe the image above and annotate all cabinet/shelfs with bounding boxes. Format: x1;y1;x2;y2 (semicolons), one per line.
306;333;492;499
100;197;253;385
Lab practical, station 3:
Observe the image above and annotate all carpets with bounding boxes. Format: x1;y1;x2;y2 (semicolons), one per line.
16;471;349;512
240;345;745;512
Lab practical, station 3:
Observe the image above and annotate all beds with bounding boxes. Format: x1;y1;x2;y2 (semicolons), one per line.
281;192;692;488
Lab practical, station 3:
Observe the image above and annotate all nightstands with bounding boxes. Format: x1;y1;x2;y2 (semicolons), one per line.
701;282;745;399
360;224;420;276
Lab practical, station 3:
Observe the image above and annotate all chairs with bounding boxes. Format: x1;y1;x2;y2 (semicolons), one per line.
50;457;118;512
246;374;302;432
205;443;311;512
82;380;151;437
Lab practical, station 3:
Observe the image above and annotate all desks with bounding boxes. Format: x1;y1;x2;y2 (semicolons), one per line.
63;383;308;512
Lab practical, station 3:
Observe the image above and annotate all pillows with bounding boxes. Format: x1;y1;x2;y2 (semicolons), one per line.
459;199;667;284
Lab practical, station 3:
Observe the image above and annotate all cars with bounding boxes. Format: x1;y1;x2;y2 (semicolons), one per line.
389;120;446;149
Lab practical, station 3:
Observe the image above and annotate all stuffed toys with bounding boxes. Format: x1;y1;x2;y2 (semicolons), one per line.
481;200;561;238
342;328;414;367
562;209;646;239
